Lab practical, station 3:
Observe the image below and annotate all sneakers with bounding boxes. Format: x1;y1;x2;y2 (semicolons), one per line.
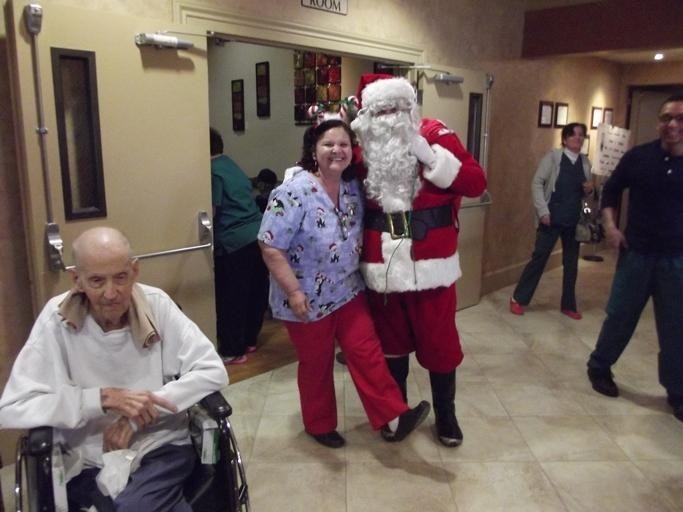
388;399;432;442
560;306;583;321
586;365;620;398
303;428;348;450
508;295;527;317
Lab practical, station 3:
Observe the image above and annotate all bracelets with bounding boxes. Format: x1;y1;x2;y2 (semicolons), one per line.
284;287;302;296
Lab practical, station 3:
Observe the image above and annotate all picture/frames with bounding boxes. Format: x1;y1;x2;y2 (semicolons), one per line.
537;100;553;128
590;106;603;129
230;79;245;131
553;102;568;128
255;61;269;117
603;107;612;125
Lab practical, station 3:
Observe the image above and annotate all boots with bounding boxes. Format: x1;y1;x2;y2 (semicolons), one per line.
425;370;465;448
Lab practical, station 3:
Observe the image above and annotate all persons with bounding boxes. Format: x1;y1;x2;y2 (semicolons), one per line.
349;73;487;447
253;168;277;214
210;126;264;364
257;120;430;448
0;225;232;512
587;95;683;422
509;122;593;319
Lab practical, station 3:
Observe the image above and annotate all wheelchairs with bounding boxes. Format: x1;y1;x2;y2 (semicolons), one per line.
10;302;252;511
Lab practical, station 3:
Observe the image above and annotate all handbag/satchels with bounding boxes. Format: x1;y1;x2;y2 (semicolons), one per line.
574;214;605;245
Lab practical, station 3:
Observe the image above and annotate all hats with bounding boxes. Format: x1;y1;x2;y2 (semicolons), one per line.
355;73;417;111
